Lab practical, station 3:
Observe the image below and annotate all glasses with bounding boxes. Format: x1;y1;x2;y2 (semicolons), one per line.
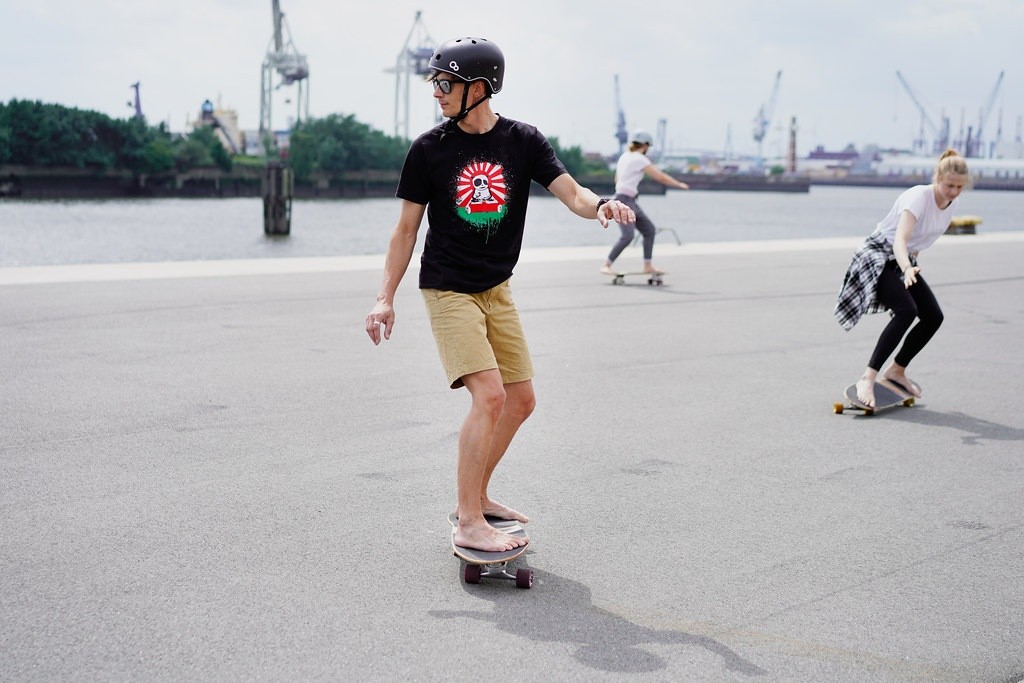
431;79;468;94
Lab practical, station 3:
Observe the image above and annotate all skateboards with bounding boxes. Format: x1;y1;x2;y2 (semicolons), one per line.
601;271;669;285
833;377;922;415
447;511;534;588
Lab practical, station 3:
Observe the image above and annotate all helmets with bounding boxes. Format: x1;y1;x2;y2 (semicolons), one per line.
429;38;505;94
632;132;653;147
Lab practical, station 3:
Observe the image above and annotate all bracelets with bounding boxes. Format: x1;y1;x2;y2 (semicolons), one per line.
595;197;610;213
901;266;915;275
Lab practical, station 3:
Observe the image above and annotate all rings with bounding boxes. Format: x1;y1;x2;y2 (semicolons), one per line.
373;322;381;325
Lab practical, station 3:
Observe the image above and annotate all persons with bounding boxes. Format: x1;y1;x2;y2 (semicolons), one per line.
366;36;635;552
833;150;971;408
600;132;690;274
